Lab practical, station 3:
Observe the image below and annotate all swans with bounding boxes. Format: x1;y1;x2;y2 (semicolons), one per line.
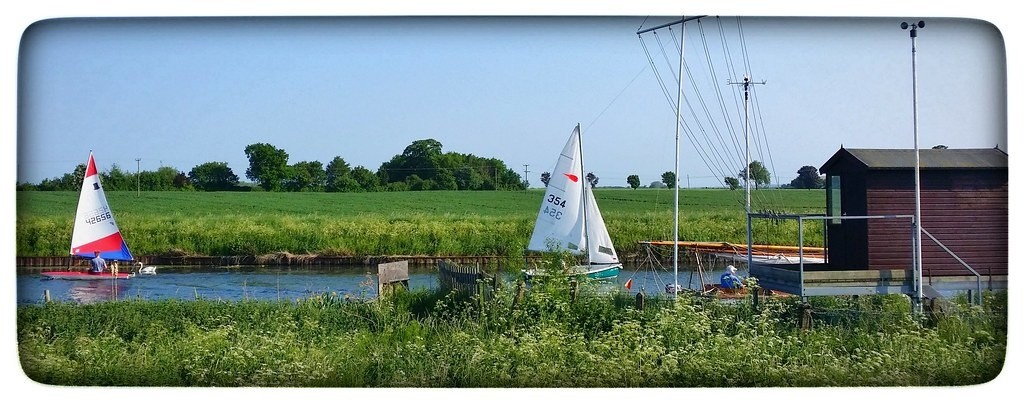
138;261;157;275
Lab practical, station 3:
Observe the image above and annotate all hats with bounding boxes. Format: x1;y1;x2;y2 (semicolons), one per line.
726;265;737;274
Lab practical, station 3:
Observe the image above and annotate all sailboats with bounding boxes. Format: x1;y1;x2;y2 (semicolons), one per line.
521;123;624;284
40;149;136;281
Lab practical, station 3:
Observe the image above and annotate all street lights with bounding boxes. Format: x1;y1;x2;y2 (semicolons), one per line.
900;20;927;320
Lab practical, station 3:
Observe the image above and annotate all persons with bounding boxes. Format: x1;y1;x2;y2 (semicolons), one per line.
721;265;746;289
86;251;107;273
